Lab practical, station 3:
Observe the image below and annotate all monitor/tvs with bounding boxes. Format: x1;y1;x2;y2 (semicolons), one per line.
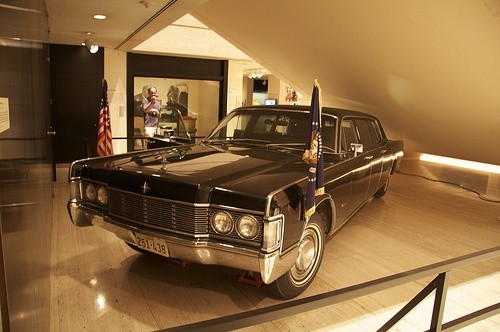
265;99;276;105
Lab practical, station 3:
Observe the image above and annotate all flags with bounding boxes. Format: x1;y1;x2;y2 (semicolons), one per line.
302;83;324;217
96;161;113;169
96;80;114;158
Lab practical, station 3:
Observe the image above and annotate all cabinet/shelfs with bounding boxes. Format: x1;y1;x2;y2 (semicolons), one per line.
133;116;147;151
177;116;196;145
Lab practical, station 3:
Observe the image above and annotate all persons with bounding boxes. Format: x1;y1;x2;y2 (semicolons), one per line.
134;85;188;123
142;87;161;137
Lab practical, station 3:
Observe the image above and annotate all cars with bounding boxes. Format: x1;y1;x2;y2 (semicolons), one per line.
67;79;403;300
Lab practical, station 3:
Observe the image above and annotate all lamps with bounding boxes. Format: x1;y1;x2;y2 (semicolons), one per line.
82;38;98;53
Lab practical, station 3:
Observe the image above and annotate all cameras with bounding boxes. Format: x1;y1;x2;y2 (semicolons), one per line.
153;94;158;97
